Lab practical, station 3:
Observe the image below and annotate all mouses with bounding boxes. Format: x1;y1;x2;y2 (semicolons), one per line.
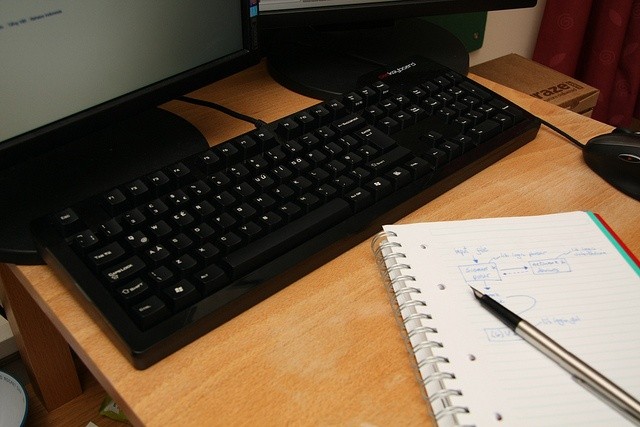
580;131;639;205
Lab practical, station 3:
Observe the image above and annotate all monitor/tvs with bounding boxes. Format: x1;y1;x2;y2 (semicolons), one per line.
257;1;538;100
1;0;250;149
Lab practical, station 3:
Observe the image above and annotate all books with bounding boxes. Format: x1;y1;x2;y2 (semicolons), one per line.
376;209;639;427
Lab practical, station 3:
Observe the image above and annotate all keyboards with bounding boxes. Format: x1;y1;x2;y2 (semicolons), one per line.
29;53;542;374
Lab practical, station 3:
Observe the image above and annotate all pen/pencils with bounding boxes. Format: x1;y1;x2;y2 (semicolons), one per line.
470;287;640;427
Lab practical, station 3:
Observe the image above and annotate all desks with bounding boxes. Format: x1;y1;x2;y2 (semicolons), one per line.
0;68;640;427
